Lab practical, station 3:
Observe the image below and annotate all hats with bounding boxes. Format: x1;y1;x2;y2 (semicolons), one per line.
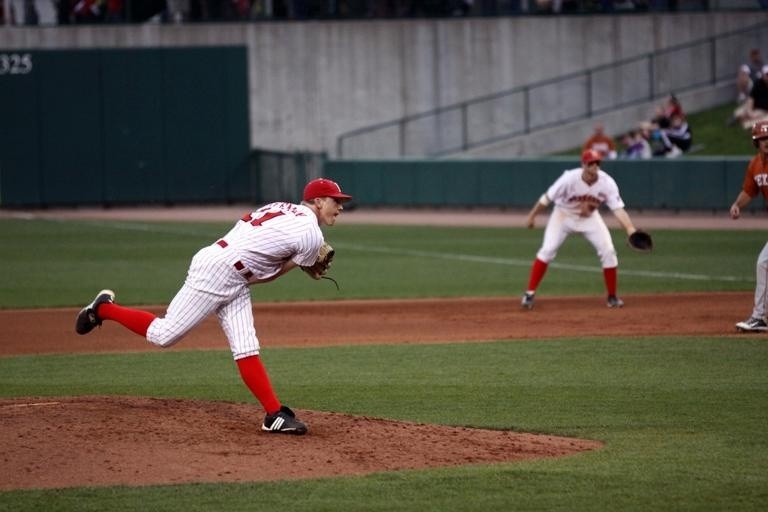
302;177;351;203
580;148;603;164
751;119;768;141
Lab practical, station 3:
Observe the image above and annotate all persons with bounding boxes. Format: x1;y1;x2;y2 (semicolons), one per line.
582;92;691;160
724;48;768;132
520;150;654;307
729;120;768;331
75;177;353;434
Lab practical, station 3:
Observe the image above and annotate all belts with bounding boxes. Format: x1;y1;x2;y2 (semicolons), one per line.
216;239;257;285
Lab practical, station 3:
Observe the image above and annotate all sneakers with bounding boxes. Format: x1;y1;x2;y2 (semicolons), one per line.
74;288;116;335
734;316;768;332
520;292;535;310
605;295;626;308
259;405;308;435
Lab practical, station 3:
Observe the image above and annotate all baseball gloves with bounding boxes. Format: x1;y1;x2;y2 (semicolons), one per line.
299;242;335;281
625;228;654;255
